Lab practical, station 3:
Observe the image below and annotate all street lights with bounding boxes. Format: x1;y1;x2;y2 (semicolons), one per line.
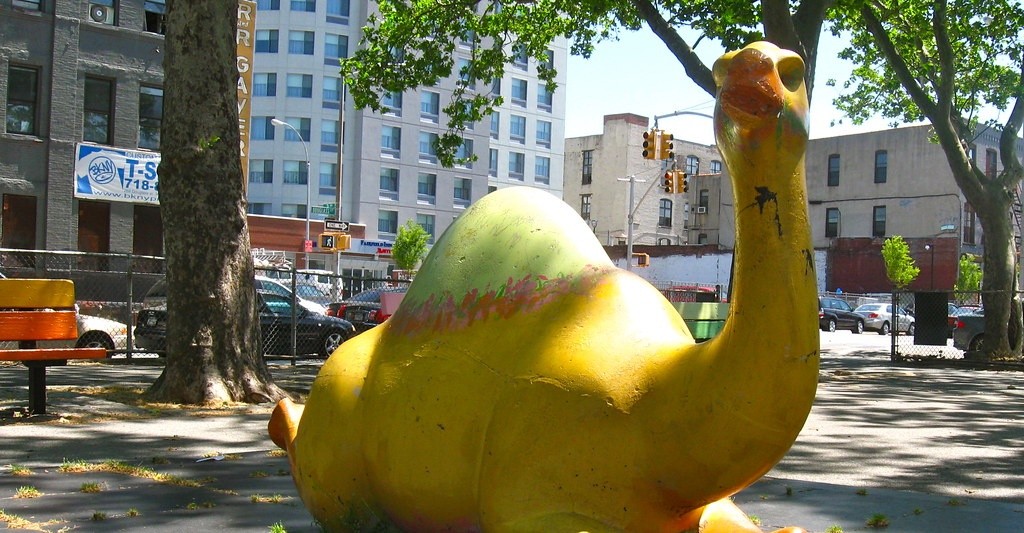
270;118;312;270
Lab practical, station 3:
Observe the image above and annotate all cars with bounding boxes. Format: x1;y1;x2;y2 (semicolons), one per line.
0;271;132;360
327;285;411;335
134;292;355;359
289;282;329;298
905;301;957;334
951;304;990;352
948;307;980;338
664;286;727;302
854;302;915;336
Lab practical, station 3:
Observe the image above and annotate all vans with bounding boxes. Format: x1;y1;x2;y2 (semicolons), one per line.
135;274;323;318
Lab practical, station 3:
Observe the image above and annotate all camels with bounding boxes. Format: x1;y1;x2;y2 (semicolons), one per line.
266;37;821;533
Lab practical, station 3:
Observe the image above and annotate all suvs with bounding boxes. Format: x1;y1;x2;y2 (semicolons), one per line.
819;297;865;334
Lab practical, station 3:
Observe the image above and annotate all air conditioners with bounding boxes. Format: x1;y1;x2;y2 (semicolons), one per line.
698;207;706;213
88;4;114;25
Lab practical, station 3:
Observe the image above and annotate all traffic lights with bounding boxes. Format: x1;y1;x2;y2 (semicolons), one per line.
664;171;675;194
637;252;650;268
660;131;674;160
678;172;688;193
320;234;334;251
642;131;656;160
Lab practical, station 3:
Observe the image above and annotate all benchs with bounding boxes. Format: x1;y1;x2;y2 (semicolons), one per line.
669;302;730;339
0;278;106;415
